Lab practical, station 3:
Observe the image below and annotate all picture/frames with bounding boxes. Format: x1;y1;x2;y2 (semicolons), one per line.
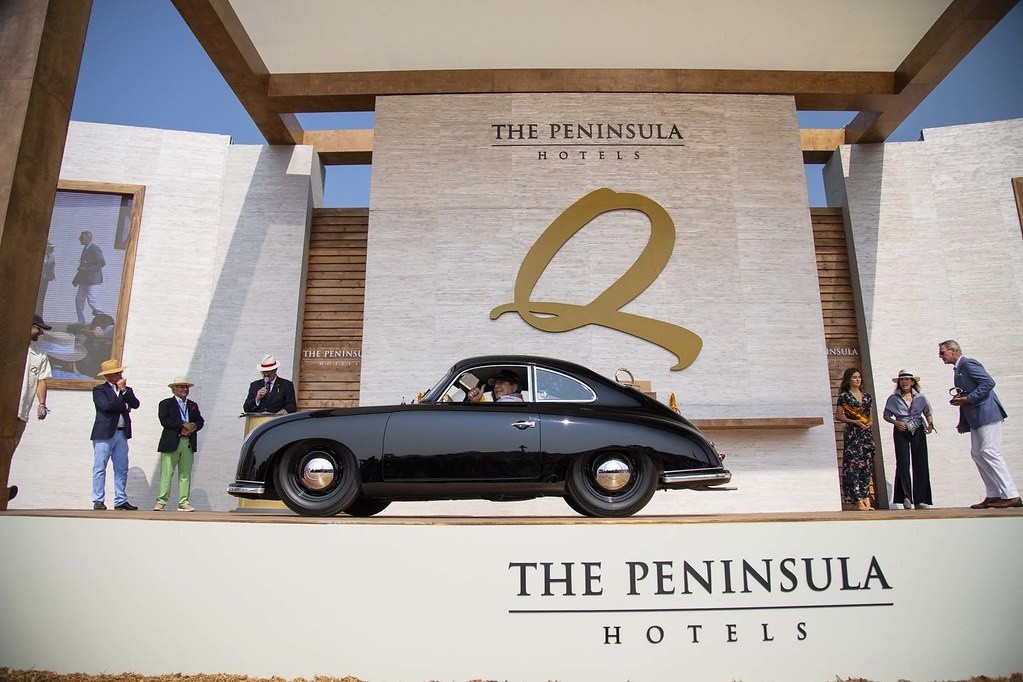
31;178;147;391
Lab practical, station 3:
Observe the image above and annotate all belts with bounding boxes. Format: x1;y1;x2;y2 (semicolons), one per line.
117;427;126;430
180;434;186;437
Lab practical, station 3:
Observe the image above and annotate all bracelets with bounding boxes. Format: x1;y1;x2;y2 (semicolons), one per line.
38;402;45;408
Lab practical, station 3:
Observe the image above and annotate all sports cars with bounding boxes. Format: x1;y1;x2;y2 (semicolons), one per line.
220;353;738;520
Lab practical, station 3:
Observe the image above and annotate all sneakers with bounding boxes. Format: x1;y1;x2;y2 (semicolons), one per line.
153;503;165;511
178;502;196;512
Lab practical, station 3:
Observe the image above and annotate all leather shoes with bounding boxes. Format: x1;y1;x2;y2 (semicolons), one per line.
114;501;138;510
987;497;1023;507
971;497;1000;508
94;502;107;510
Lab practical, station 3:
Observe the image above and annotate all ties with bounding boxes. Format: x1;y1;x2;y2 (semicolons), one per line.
267;381;272;394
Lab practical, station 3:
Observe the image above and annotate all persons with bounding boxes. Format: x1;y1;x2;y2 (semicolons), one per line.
8;314;53;502
883;369;934;509
443;369;524;402
153;376;205;512
34;239;55;319
939;340;1022;509
72;231;105;323
90;359;140;510
835;368;876;511
34;315;115;380
243;354;296;415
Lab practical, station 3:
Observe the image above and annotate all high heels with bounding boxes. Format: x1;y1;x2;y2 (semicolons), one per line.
854;498;875;511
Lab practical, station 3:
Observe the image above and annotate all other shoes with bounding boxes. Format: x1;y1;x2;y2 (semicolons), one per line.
914;503;928;509
904;502;911;509
7;485;18;501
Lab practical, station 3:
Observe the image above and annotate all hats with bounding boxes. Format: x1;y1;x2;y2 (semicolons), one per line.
31;314;52;330
168;376;194;388
257;355;281;371
488;369;522;387
892;369;920;383
97;359;127;376
46;242;55;248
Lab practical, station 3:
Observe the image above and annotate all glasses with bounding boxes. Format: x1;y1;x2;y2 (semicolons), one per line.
32;325;42;331
940;348;958;355
